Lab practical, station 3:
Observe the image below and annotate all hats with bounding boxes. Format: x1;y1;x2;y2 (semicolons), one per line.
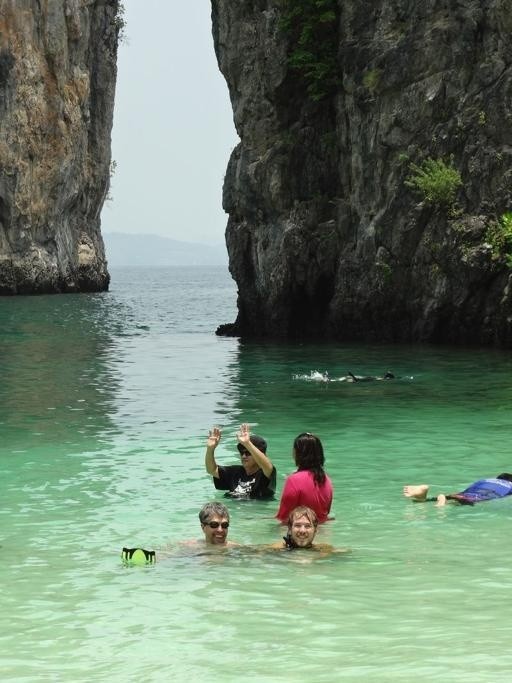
237;436;267;450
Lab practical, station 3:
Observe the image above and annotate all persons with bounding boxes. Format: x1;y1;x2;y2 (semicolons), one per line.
254;505;349;554
402;472;512;507
206;423;277;500
155;501;241;561
274;432;333;529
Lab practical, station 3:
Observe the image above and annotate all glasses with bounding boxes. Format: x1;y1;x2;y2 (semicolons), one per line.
206;521;229;528
240;450;251;456
